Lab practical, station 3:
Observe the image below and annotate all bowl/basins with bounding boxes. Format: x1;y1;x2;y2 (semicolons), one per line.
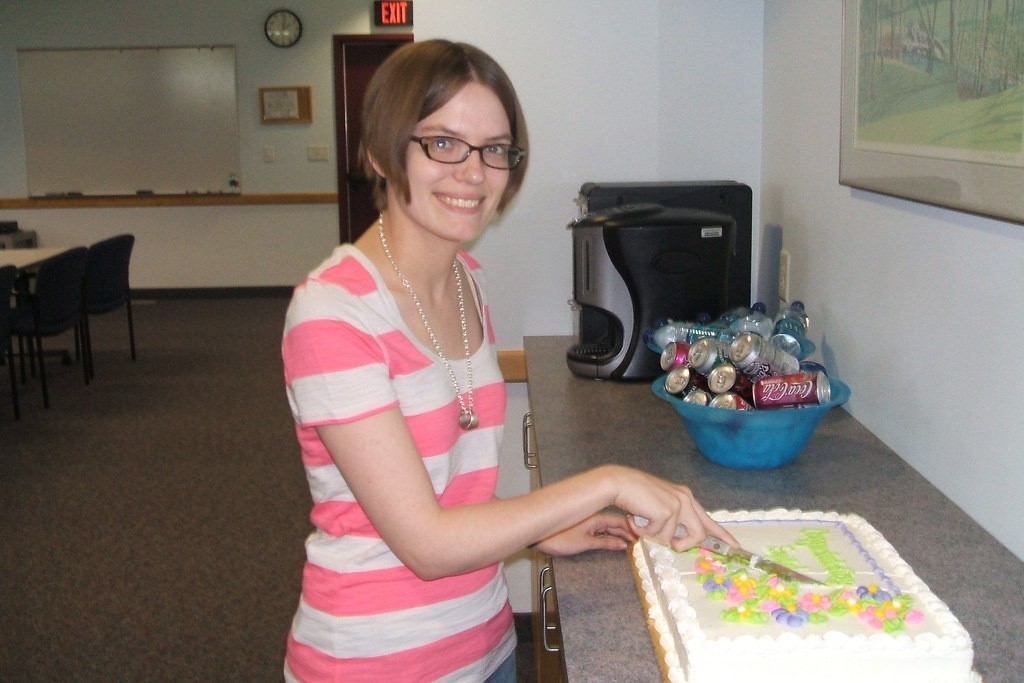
650;374;850;470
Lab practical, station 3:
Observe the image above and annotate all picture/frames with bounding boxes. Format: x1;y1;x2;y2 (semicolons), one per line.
838;0;1024;225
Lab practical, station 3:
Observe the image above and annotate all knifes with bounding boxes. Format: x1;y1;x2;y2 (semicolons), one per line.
635;515;831;585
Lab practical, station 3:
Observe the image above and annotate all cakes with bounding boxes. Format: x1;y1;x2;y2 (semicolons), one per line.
634;512;983;683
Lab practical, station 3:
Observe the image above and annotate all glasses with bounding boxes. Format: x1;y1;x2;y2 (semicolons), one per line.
409;136;526;170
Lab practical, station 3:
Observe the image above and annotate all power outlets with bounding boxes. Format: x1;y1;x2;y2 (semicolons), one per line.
779;251;789;304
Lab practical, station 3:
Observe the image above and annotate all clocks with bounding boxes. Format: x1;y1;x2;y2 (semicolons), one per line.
265;9;303;48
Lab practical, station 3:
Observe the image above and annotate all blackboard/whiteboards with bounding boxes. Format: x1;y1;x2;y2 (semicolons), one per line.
16;44;241;199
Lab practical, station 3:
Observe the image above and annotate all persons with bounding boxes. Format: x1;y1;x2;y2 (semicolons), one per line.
280;38;741;683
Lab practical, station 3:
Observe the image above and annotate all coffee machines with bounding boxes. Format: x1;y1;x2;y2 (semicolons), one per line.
566;180;752;379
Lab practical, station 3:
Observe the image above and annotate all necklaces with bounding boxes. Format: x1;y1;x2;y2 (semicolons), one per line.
377;212;473;413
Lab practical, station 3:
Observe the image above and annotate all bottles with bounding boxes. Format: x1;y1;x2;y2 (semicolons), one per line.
644;302;810;356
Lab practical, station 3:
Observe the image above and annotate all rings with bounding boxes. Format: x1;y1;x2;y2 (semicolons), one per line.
458;410;479;429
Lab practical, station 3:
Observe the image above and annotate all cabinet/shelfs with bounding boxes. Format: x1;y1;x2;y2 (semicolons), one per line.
522;335;1024;683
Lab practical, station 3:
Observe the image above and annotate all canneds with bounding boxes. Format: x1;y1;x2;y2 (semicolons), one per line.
659;333;832;412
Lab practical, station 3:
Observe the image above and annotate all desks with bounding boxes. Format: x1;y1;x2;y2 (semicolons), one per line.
0;248;79;360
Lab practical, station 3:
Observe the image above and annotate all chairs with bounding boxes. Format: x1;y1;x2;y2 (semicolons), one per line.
0;246;91;421
74;234;137;361
